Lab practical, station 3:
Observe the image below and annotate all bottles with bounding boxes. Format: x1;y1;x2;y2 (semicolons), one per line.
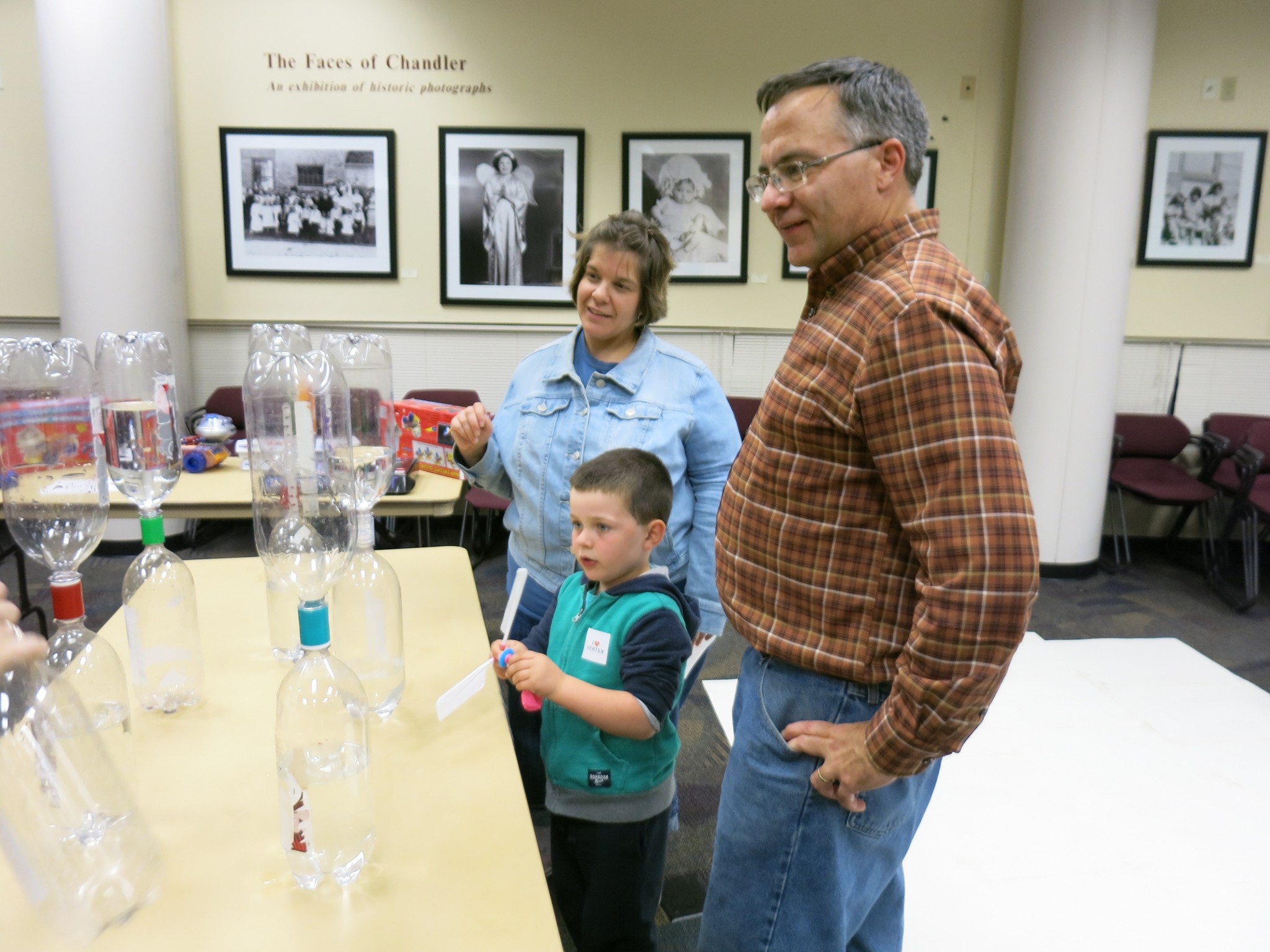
0;321;419;927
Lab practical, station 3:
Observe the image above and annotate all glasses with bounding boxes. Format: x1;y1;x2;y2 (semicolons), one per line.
746;142;880;202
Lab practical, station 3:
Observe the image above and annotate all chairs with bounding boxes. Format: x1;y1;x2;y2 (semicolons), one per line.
1100;412;1218;573
1201;411;1270;610
177;375;510;551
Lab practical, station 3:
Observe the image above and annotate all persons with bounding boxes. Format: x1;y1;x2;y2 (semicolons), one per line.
694;52;1047;952
479;150;526;284
487;445;708;952
240;179;374;243
650;174;727;262
0;578;55;679
450;209;745;832
1166;181;1235;246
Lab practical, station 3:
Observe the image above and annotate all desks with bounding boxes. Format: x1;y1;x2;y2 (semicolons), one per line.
2;548;566;948
0;448;465;640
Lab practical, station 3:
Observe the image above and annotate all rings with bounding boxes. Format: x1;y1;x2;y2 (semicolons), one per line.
817;766;833;786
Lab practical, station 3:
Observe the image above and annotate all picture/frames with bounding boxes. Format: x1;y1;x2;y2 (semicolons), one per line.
218;124;398;279
439;125;586;308
1134;125;1269;266
781;146;937;281
620;129;752;283
